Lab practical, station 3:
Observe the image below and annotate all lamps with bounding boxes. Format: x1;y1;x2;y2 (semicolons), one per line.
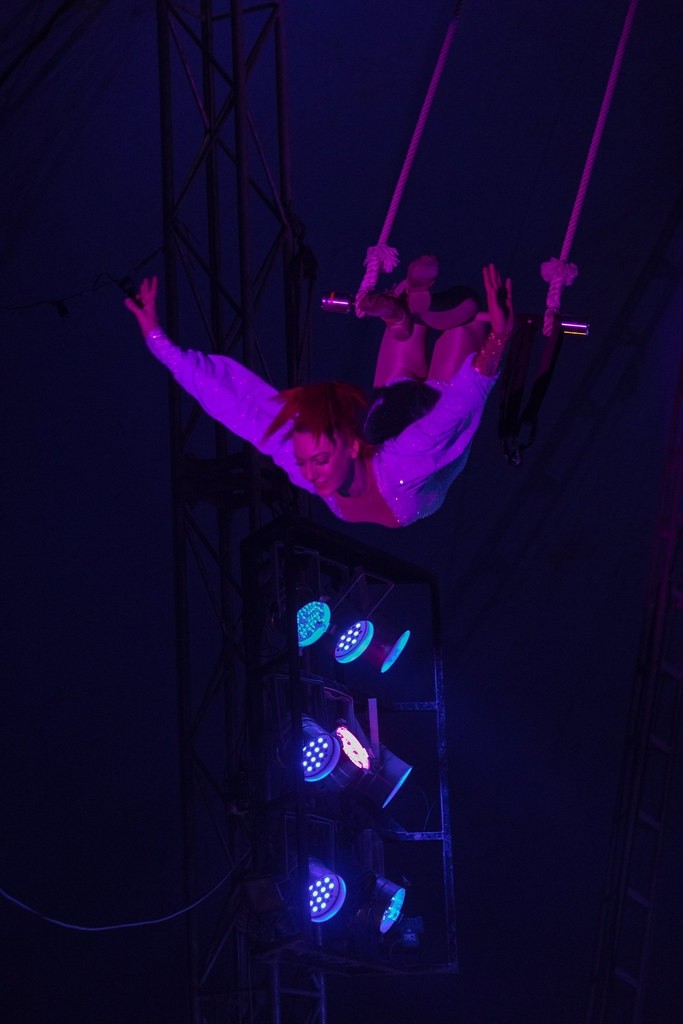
267;545;411;932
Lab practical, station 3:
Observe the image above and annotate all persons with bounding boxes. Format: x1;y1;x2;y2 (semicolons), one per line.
123;262;517;527
